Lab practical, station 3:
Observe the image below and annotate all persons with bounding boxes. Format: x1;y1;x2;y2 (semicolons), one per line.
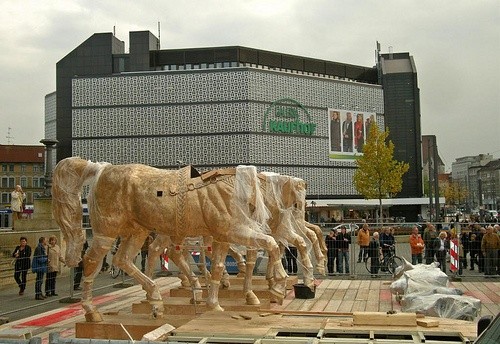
342;112;353;152
278;236;299;276
133;227;163;273
75;234;89;291
11;184;26;231
45;235;66;297
365;114;375;144
101;235;127;275
330;110;341;152
305;210;500;279
12;237;32;296
353;113;364;153
31;236;51;300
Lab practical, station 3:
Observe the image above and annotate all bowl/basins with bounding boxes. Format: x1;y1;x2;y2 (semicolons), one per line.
293;283;316;299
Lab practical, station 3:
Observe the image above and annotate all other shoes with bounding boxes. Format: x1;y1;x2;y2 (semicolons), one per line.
461;265;467;269
469;268;474;271
371;274;380;278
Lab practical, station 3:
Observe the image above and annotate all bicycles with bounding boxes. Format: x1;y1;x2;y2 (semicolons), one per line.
365;242;404;276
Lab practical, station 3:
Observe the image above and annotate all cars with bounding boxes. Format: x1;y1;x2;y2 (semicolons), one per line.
333;224;359;235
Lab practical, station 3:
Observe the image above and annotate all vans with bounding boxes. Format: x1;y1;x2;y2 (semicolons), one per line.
488;210;497;218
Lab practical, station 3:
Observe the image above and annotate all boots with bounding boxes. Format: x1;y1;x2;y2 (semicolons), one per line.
73;283;82;290
141;265;145;273
35;292;47;300
45;289;58;297
19;284;26;295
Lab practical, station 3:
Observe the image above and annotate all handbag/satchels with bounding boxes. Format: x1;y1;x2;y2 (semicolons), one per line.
11;259;17;266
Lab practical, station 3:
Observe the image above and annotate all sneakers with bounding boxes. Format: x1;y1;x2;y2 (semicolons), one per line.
357;259;361;263
363;259;366;262
